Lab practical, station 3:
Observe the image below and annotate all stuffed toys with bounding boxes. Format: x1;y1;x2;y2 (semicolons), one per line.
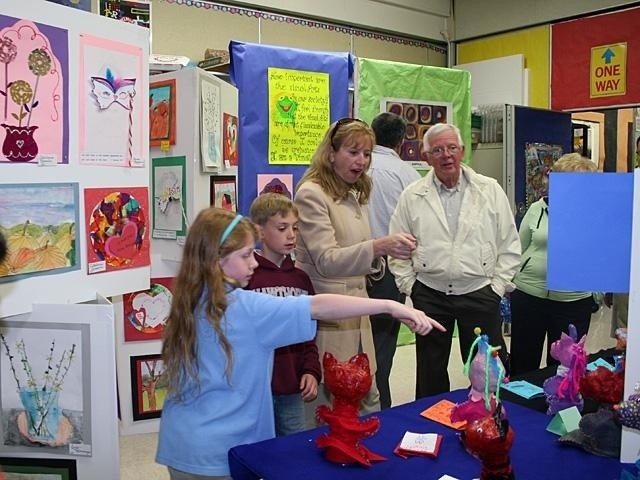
543;332;588;416
451;327;507;440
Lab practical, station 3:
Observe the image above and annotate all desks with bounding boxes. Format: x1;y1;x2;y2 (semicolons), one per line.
491;345;625;439
227;387;640;480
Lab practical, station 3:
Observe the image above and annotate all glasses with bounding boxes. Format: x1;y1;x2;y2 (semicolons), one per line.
422;144;463;155
330;117;369;150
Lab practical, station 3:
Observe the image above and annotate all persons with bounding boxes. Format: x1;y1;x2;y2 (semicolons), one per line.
154;207;448;480
362;113;422;409
385;122;522;400
240;192;323;440
506;153;606;375
291;116;417;420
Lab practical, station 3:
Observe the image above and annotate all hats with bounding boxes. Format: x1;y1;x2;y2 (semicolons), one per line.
556;409;621;458
